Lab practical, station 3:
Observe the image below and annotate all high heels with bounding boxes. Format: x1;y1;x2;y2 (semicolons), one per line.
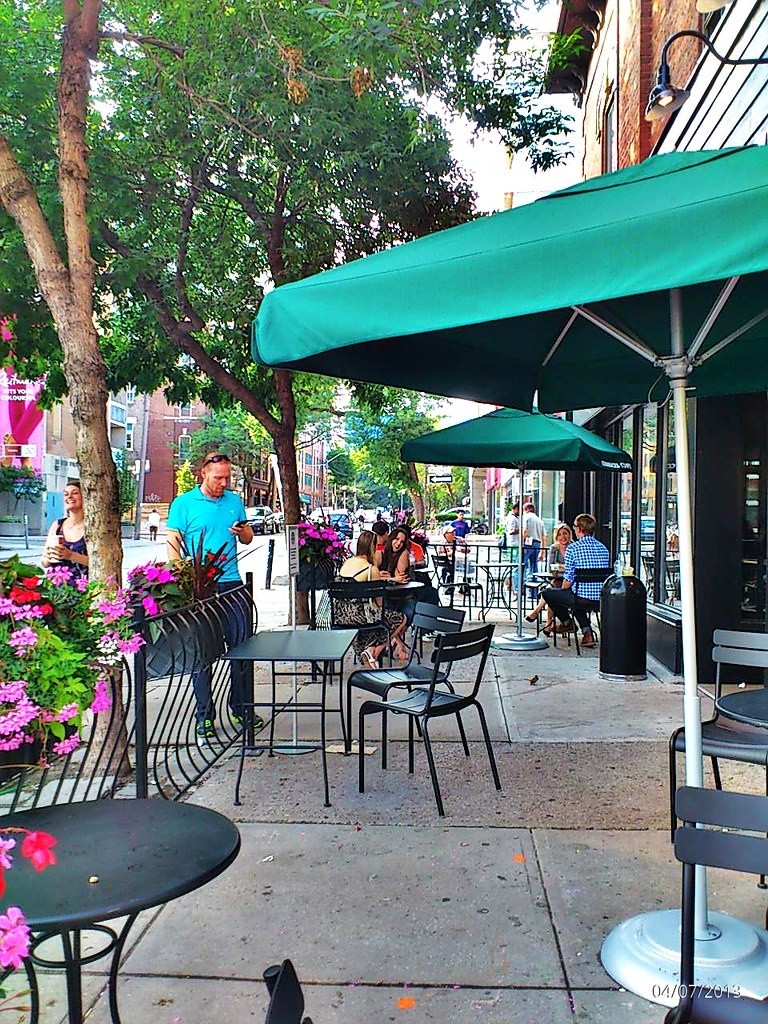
525;613;538;623
543;626;551;636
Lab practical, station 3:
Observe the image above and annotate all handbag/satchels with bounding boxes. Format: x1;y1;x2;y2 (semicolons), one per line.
362;564;381;623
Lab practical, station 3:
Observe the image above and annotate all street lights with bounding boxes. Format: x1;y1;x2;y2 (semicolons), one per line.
354;464;371;514
326;452;348;507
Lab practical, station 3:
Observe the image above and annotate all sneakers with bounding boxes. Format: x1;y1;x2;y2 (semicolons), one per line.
230;711;265;728
196;719;215;737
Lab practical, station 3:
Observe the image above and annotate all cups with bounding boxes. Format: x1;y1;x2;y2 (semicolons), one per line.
48;534;63;563
552;564;559;576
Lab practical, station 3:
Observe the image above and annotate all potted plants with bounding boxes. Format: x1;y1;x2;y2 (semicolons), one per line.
0;515;26;537
120;521;135;539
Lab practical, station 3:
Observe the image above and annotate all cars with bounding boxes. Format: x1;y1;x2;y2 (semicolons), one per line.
309;507;377;540
620;512;656;541
245;505;285;535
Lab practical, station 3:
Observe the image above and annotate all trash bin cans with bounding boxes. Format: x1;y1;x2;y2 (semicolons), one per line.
597;575;648;683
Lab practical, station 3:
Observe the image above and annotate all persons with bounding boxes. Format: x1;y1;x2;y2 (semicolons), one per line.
542;514;609;647
505;500;546;601
377;510;382;522
438;510;470;595
164;452;264;738
335;522;439;668
525;523;574;635
41;480;89;601
358;515;365;529
149;510;160;541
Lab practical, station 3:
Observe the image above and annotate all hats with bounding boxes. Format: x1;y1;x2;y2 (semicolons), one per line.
442;525;456;535
359;505;363;508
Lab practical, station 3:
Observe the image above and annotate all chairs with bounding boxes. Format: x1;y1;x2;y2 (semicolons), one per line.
664;787;768;1024
261;961;312;1024
346;601;470;758
520;547;551;623
323;582;392;686
553;568;614;654
431;554;484;608
357;623;503;818
668;630;768;889
641;551;680;607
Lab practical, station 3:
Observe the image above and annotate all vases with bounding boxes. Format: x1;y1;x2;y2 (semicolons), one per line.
142;580;253;676
296;557;335;590
0;707;79;787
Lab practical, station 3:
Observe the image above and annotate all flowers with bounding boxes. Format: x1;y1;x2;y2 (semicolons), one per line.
294;505;355;572
0;505;264;976
380;509;436;555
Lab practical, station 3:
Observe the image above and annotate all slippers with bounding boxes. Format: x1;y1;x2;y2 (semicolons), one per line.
580;638;597;646
551;624;578;634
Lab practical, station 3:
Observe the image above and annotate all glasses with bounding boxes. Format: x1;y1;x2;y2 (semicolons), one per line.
203;454;230;468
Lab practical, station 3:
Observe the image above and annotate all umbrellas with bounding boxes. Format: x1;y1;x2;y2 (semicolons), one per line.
399;405;635;650
249;140;768;1006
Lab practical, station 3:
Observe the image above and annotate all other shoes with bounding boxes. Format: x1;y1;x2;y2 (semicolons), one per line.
444;586;455;595
459;588;471;596
410;629;434;642
359;648;379;669
527;596;541;602
513;589;524;602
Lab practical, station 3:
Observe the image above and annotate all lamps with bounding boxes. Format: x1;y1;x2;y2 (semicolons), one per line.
643;30;768;122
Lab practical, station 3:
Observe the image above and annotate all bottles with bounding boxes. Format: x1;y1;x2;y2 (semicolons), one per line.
409;554;415;570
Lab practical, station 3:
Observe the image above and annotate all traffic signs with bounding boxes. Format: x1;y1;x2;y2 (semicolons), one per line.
430;475;452;483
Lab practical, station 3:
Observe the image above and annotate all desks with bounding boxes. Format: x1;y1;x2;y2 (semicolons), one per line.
469;563;524;623
328;580;425;623
222;629;360;805
1;785;242;1024
533;573;563;609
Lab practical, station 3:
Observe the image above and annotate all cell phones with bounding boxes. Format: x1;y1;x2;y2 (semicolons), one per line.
235;519;250;528
